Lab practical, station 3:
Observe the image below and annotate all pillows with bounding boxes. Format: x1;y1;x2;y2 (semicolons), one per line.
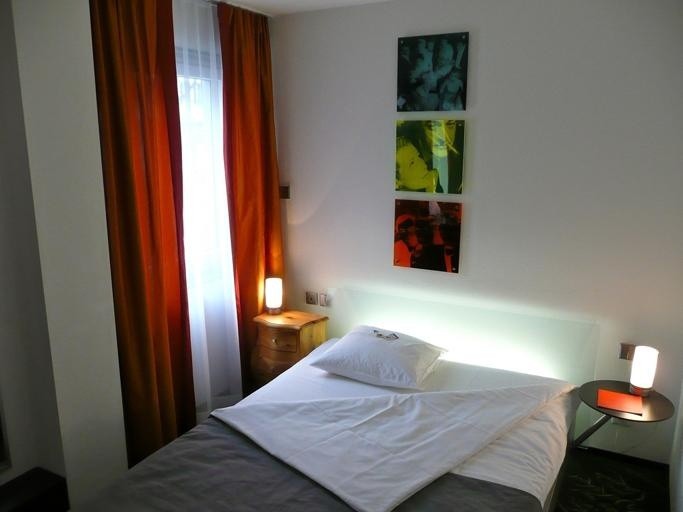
309;322;446;393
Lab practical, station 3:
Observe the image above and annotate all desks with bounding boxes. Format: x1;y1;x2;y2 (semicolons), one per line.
570;380;677;444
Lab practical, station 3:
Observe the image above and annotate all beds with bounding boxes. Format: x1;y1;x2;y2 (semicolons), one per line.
67;337;581;507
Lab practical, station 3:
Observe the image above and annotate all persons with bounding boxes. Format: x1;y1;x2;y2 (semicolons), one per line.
395;34;464;273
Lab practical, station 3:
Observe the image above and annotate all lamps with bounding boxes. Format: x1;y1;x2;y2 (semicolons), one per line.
264;278;282;314
629;344;660;397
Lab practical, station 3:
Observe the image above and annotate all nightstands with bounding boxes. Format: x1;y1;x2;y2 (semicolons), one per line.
250;309;327;384
